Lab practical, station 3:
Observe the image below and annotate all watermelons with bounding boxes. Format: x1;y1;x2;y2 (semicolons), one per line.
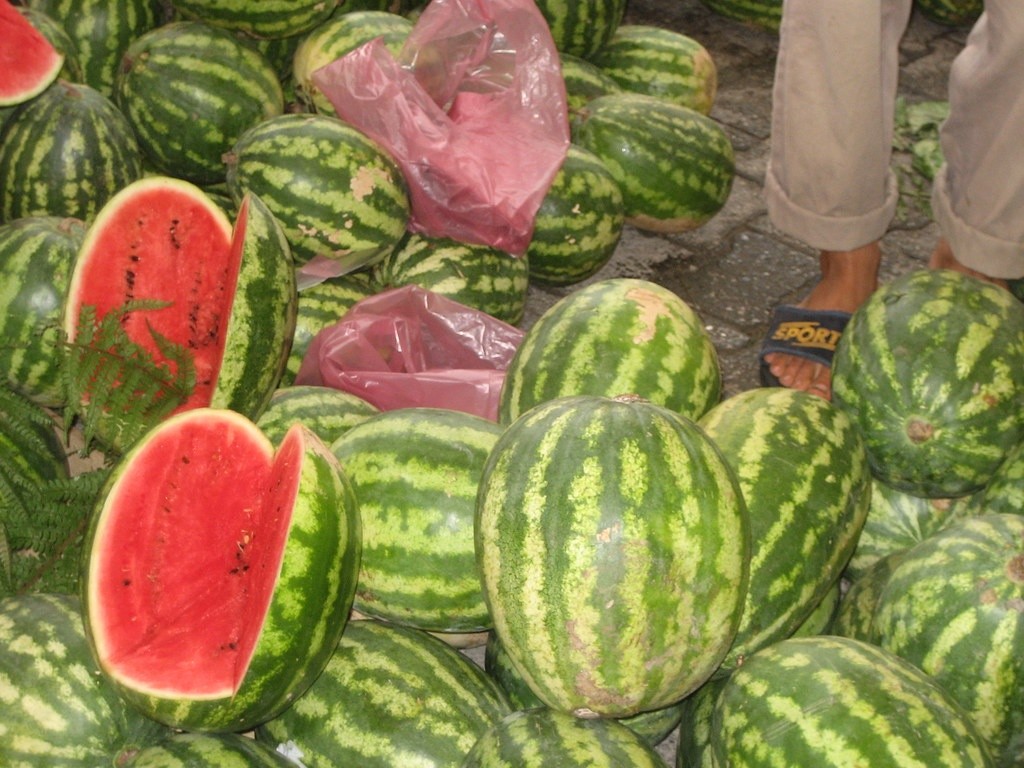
0;0;1023;768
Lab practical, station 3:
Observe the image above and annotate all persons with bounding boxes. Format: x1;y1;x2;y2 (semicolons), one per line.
759;0;1024;403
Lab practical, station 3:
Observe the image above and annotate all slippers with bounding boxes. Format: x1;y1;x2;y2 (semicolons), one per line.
758;302;854;405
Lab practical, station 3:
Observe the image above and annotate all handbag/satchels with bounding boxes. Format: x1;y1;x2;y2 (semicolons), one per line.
292;285;530;424
311;0;571;260
